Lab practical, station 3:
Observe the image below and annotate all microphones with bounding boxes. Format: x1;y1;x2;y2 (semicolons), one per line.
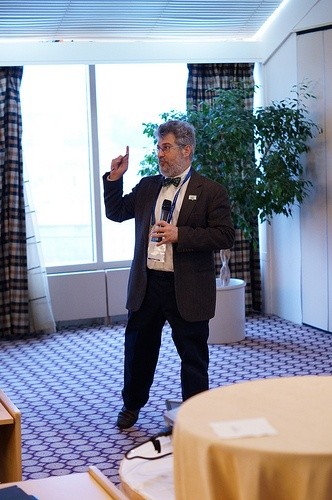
156;199;171;242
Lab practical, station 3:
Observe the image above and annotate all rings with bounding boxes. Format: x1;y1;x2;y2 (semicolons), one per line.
163;233;164;236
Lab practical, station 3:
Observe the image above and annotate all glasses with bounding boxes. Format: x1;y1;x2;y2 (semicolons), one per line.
154;145;184;153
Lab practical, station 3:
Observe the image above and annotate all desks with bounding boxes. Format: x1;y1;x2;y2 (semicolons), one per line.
174;375;332;500
0;465;130;500
0;388;21;485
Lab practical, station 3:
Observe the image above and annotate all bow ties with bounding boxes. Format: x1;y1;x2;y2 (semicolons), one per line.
161;177;180;187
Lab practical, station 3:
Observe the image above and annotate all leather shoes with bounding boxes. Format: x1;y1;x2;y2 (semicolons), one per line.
117;395;151;430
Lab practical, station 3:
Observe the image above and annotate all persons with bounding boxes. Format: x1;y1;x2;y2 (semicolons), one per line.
102;120;236;429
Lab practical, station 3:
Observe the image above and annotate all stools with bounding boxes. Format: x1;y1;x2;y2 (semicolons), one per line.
119;436;174;500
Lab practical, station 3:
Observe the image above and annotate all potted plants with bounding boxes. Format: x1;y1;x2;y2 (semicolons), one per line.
138;79;324;344
